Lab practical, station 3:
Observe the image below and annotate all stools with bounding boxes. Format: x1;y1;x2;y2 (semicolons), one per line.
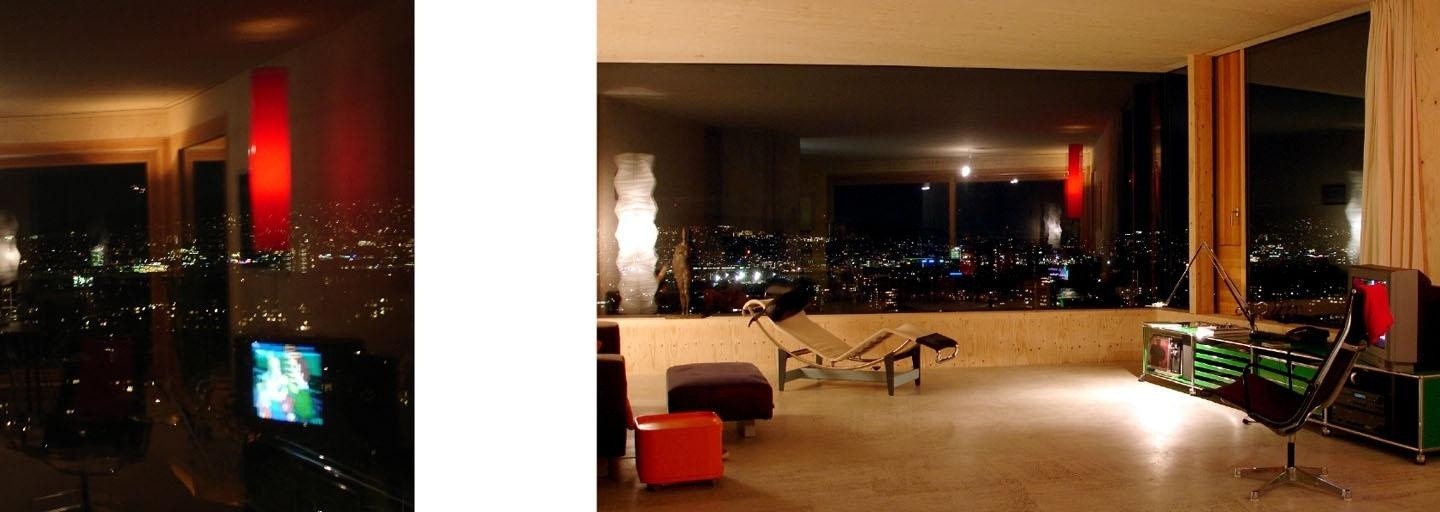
667;363;772;437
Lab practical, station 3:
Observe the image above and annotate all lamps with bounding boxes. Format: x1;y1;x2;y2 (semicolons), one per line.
615;151;660;315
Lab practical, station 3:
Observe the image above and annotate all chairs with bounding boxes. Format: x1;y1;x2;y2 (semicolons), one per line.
44;358;149;512
742;285;960;396
597;319;626;478
1189;288;1373;502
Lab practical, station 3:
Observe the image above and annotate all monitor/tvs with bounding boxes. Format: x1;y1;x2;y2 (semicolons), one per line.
1346;264;1440;372
231;331;373;448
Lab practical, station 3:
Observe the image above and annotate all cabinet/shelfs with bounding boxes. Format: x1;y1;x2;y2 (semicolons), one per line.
240;418;414;512
1140;323;1440;463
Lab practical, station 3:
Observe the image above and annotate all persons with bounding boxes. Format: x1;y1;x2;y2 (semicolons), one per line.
1150;337;1167;366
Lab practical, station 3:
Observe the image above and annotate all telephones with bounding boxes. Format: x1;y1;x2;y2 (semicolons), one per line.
1286;326;1329;343
